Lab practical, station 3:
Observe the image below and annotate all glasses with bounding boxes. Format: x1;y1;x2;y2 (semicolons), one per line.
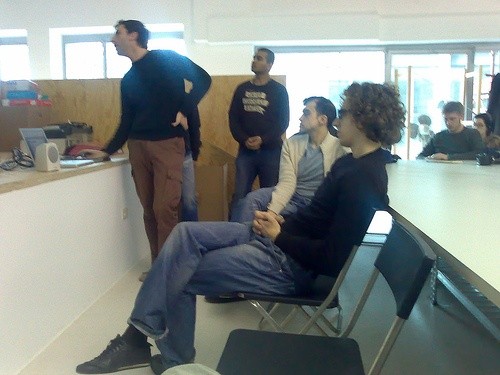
337;108;356;120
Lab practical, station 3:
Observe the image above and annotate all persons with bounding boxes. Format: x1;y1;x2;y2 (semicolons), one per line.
203;96;347;304
410;73;500;164
76;82;405;375
179;92;203;221
228;48;290;223
77;20;211;285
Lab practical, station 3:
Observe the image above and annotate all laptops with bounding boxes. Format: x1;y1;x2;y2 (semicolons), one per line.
18;127;94;168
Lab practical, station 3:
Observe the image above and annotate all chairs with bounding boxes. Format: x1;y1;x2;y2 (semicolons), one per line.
215;194;437;375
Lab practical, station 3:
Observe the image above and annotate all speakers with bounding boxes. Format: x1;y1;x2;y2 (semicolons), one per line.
35;143;60;172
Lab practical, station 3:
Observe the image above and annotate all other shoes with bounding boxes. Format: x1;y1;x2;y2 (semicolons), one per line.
138;271;150;282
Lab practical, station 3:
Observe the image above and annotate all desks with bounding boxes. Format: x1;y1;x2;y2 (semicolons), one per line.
385;158;500;342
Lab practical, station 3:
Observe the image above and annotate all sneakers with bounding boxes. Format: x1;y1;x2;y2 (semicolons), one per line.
76;333;153;374
